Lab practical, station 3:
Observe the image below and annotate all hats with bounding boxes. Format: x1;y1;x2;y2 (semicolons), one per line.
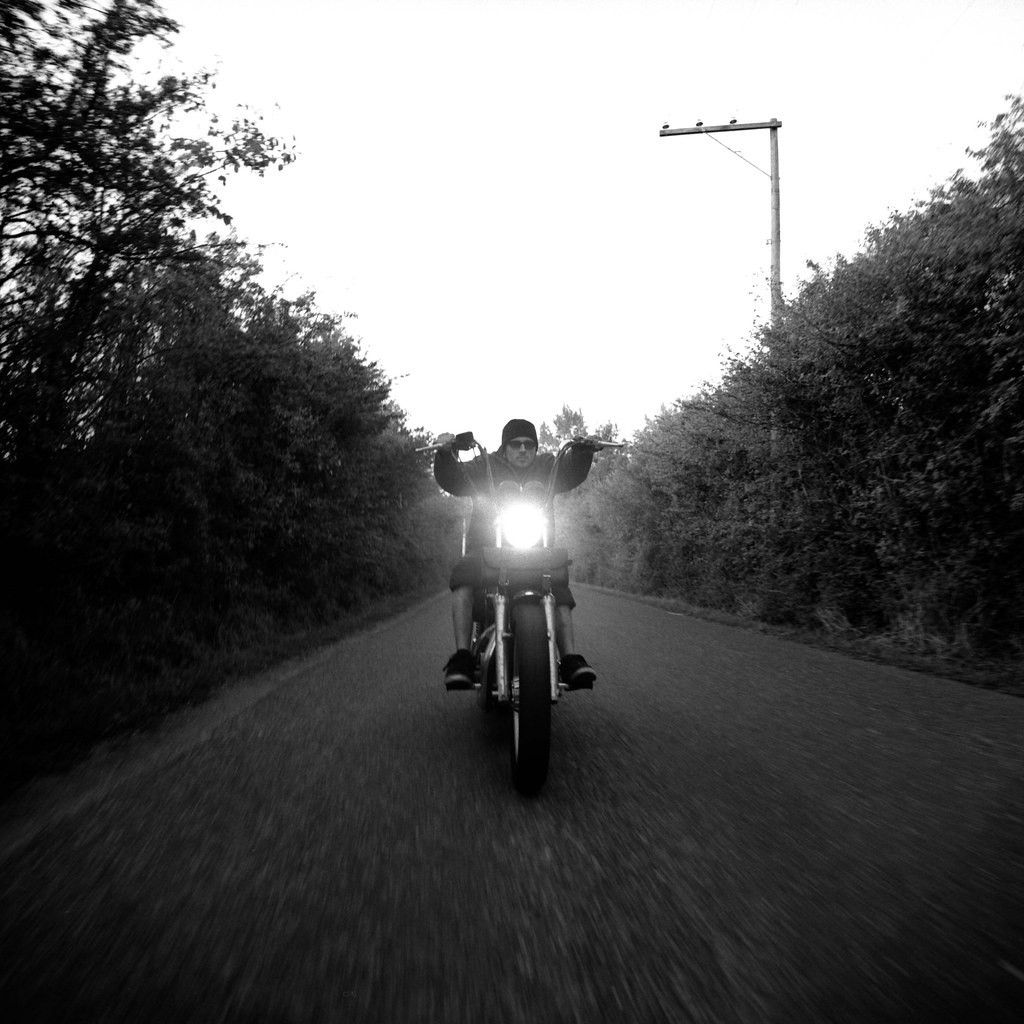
502;419;538;451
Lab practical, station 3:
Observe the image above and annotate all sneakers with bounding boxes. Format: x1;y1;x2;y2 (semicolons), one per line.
558;654;598;685
442;648;477;687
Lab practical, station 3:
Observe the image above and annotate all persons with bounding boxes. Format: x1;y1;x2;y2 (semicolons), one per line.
435;420;603;690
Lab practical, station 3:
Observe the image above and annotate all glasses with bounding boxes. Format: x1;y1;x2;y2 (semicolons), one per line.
508;440;535;450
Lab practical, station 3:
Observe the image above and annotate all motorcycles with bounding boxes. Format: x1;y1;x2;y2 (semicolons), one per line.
413;430;626;801
